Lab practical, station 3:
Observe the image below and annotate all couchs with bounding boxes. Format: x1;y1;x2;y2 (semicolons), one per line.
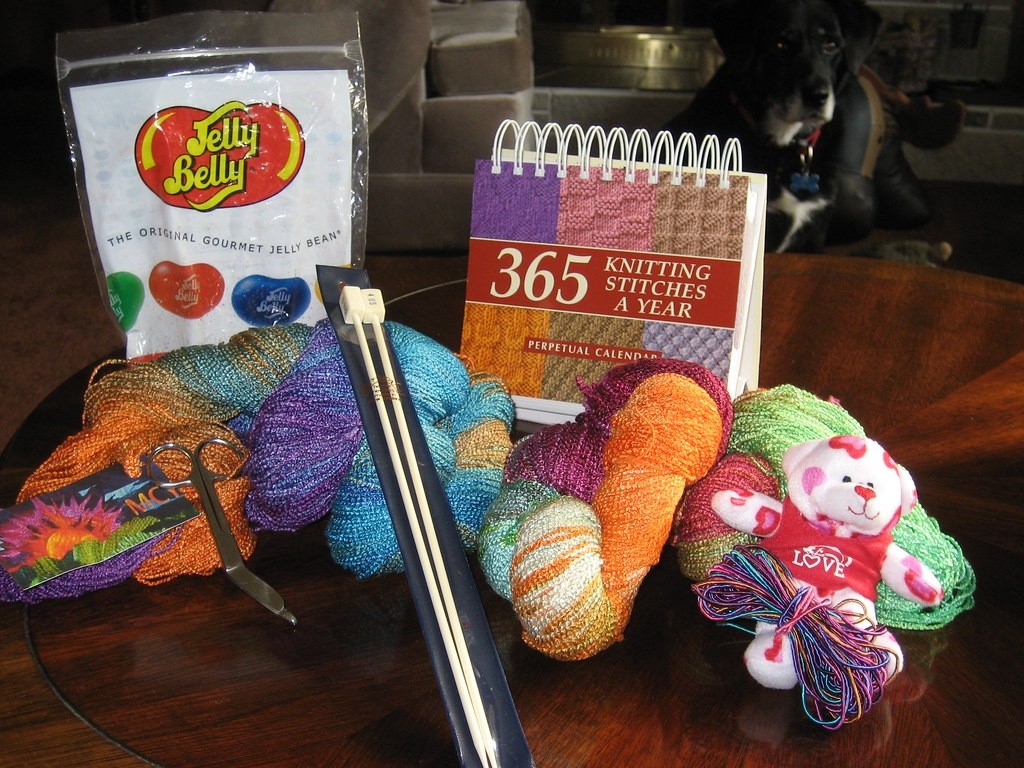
264;0;535;252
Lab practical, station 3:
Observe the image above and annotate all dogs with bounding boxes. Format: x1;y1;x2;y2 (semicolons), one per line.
634;0;884;255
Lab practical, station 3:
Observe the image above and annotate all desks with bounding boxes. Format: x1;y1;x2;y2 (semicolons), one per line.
0;256;1024;768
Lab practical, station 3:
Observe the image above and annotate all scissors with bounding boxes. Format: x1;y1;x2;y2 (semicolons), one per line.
146;436;297;626
